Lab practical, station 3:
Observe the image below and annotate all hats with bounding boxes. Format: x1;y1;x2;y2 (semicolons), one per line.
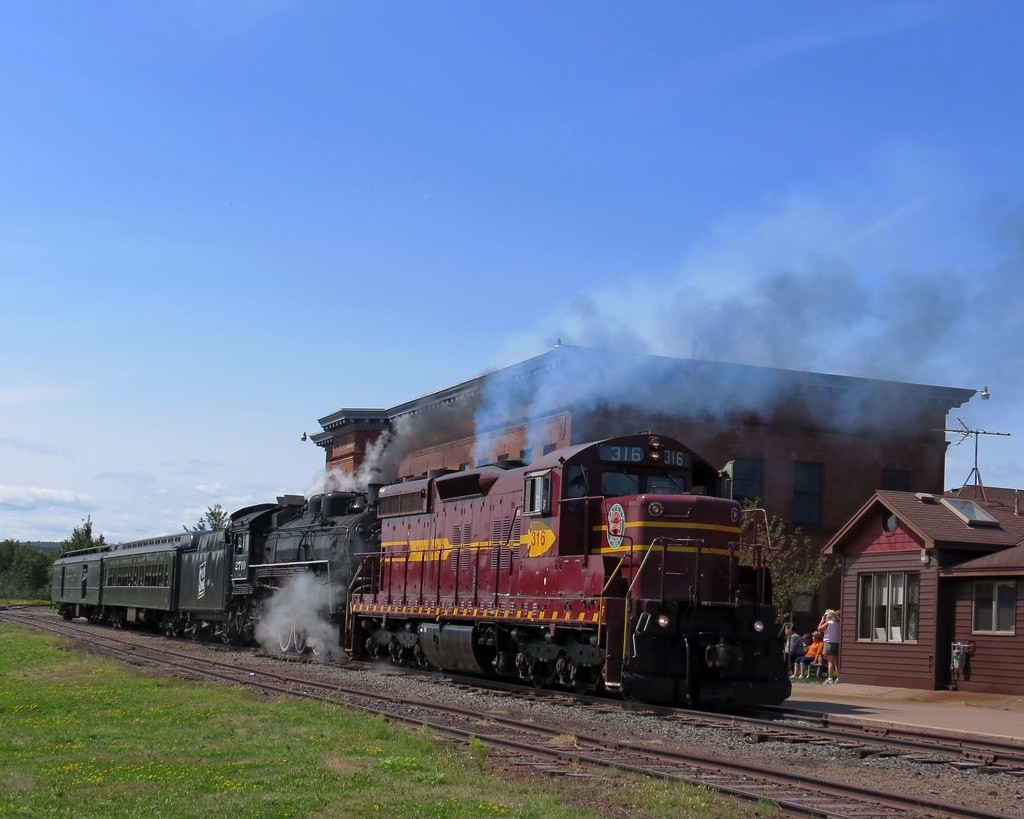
801;633;812;639
812;631;822;638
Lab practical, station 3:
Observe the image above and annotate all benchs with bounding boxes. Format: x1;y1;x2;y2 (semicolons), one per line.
810;662;826;679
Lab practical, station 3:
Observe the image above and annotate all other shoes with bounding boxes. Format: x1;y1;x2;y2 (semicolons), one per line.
823;678;833;685
789;674;797;679
798;675;803;679
835;677;839;685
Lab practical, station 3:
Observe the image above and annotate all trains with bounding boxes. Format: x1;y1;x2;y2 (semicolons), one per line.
45;427;793;709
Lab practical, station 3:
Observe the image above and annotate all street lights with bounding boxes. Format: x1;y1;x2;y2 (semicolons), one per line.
718;459;735;604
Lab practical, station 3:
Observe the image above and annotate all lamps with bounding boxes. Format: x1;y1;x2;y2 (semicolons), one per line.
976;386;989;399
554;338;562;349
301;432;310;441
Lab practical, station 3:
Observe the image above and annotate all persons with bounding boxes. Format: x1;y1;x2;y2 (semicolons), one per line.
783;610;841;685
